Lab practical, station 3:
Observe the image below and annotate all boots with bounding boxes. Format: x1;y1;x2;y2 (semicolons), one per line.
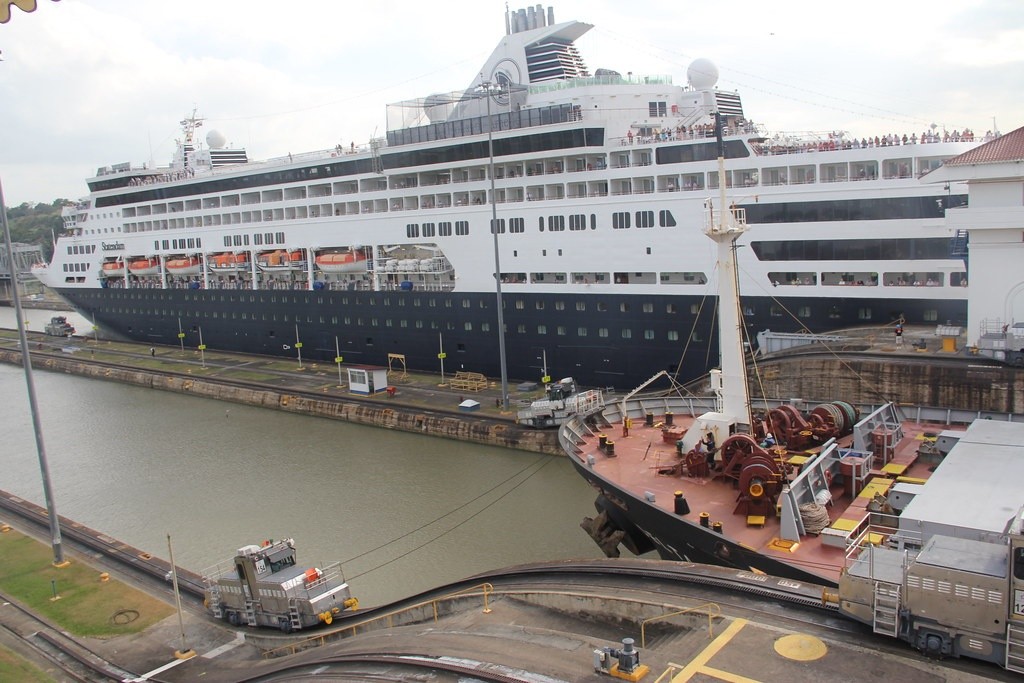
709;463;716;469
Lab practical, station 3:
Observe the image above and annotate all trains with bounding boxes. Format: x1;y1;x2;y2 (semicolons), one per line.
820;511;1024;675
202;536;359;635
515;375;606;429
44;316;76;336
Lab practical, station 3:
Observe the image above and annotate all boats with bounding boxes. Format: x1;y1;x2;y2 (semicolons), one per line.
556;110;1024;683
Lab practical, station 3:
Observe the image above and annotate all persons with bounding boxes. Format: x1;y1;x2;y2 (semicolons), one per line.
288;152;292;162
961;279;967;287
335;144;343;153
626;120;754;135
792;278;809;285
896;324;903;348
759;131;1002;155
889;277;939;286
129;168;195;186
839;278;877;285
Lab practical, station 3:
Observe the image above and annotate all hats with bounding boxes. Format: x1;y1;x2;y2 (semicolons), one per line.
707;432;712;437
896;325;900;328
767;433;773;439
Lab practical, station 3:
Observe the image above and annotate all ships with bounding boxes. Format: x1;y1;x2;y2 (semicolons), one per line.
28;0;1005;393
